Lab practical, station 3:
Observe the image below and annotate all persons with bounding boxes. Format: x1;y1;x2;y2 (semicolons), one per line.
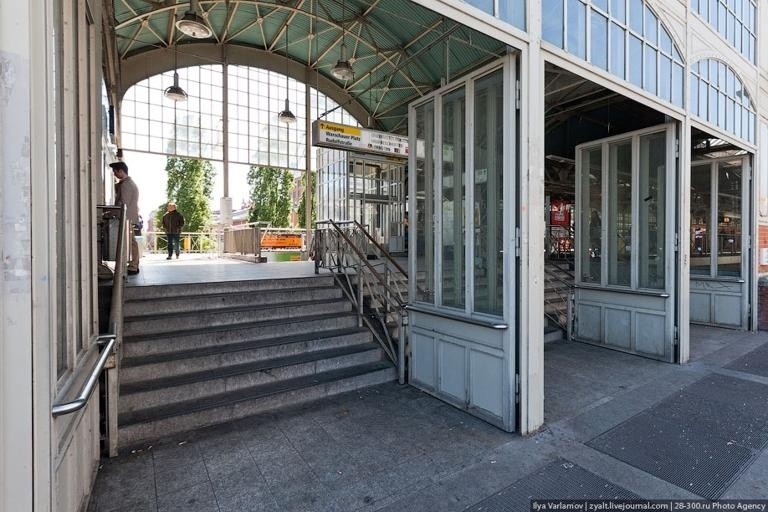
107;160;140;275
588;209;601;254
718;225;727;251
693;225;703;251
162;202;185;260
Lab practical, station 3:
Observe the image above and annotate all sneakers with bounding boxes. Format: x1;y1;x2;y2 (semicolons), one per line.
127;270;140;275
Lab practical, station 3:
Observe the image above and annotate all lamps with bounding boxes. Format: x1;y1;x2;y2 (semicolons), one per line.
331;1;355;82
277;24;295;123
162;11;189;101
176;3;213;42
